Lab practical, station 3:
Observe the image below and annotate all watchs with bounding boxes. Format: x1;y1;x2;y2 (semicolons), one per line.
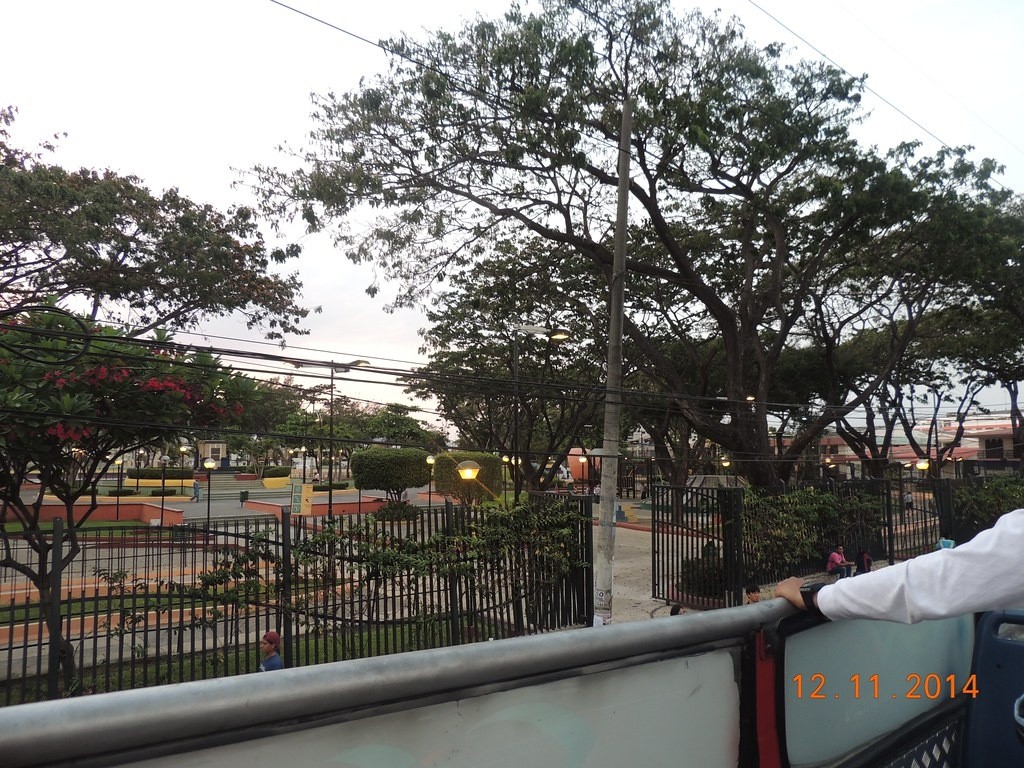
799;580;827;612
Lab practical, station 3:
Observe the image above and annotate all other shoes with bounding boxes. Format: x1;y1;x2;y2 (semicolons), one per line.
911;514;914;516
907;513;910;517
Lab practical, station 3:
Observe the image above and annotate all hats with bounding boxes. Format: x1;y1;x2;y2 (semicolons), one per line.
263;631;282;655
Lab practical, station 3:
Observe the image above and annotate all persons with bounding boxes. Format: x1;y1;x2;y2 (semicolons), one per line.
555;484;559;493
826;546;855;579
312;472;320;482
640;482;648;500
574;485;601;504
669;604;688;616
258;631;282;674
745;585;762;605
774;507;1023;625
190;476;200;503
853;553;873;577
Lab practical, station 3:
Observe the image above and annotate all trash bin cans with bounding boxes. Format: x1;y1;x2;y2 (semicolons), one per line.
239;490;248;508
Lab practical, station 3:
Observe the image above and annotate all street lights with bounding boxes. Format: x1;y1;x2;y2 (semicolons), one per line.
161;455;170;530
328;360;371;583
723;460;730;487
502;455;509;506
456;461;481;536
289;449;293;469
514;325;572;510
947;457;963;479
580;456;587;497
114;458;123;520
916;460;928;545
179;446;187;496
204;458;215;547
427;455;435;507
